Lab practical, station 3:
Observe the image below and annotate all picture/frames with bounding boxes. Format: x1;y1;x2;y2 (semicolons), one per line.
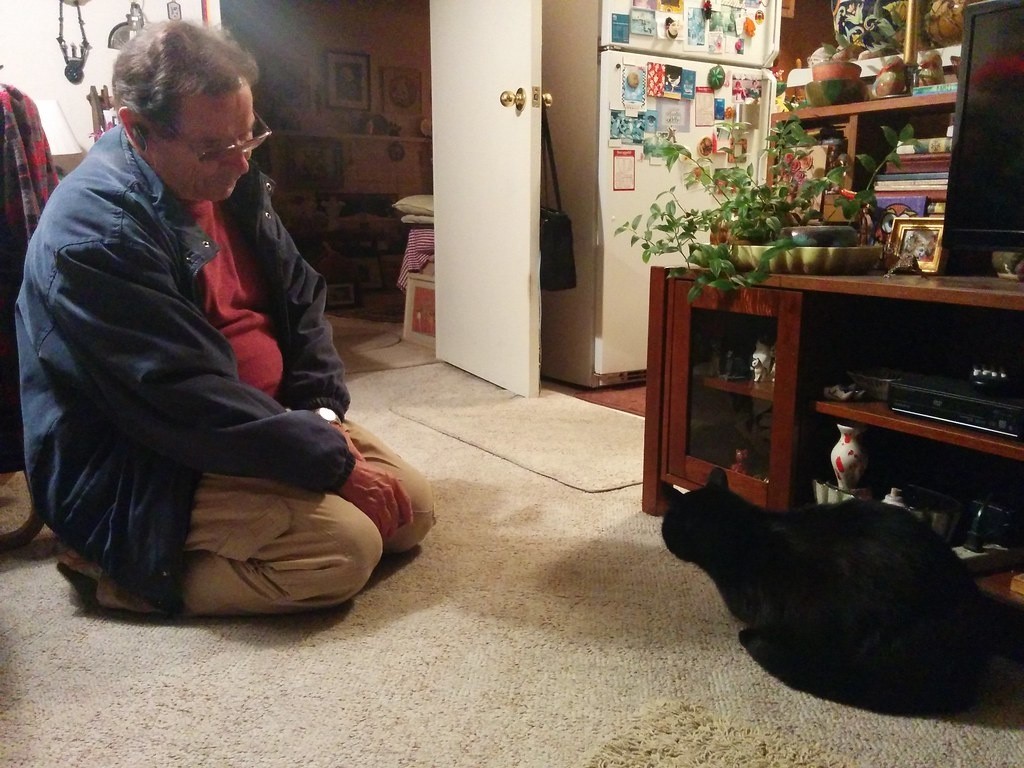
380;65;423;116
279;56;314;109
287;138;346;191
322;47;372;111
402;272;435;349
882;216;950;276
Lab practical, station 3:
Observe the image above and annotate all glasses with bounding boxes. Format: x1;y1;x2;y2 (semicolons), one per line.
138;108;274;166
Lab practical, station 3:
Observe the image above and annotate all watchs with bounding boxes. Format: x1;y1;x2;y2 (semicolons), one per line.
313;408;341;426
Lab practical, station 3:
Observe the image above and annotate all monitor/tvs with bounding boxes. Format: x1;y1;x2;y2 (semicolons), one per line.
942;0;1024;254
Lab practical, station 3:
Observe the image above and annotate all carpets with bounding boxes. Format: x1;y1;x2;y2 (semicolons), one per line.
571;383;646;416
390;389;643;493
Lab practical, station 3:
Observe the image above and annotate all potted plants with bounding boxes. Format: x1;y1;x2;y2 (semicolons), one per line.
614;93;925;303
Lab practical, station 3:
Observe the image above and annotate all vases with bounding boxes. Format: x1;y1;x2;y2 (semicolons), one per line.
831;423;868;489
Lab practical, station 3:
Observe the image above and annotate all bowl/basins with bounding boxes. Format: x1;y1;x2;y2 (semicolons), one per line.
813;62;861;82
806;78;867;106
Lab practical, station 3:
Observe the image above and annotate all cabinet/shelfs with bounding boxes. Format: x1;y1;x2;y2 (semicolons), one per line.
263;129;432;202
642;0;1024;664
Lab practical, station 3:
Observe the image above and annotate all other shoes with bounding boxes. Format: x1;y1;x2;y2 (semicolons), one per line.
54;551;111;591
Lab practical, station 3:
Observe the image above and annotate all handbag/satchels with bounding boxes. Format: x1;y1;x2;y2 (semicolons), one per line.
536;204;581;293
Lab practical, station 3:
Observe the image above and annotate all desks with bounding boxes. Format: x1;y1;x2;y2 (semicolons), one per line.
286;226;388;295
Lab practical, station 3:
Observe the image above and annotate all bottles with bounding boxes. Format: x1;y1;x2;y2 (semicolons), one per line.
830;421;868;491
880;486;905;508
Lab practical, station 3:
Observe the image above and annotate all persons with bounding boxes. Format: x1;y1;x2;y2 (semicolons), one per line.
734;81;760;100
15;21;434;614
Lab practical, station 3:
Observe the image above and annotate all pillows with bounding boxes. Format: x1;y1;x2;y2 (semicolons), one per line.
392;194;433;215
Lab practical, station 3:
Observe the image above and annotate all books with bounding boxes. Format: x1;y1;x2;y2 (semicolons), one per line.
872;137;952;218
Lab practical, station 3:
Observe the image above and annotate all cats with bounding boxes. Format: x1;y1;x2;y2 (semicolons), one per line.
661;467;1015;718
